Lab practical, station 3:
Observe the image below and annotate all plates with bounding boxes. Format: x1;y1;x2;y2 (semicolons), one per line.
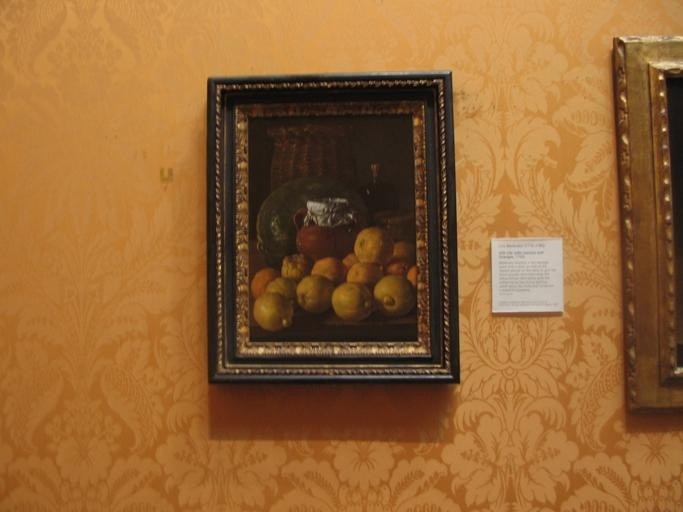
256;179;370;266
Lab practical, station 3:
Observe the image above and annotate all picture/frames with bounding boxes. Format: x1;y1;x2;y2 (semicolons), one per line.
613;34;682;412
206;69;462;386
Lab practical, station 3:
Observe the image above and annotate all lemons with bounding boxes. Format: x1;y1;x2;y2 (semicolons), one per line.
249;223;420;333
